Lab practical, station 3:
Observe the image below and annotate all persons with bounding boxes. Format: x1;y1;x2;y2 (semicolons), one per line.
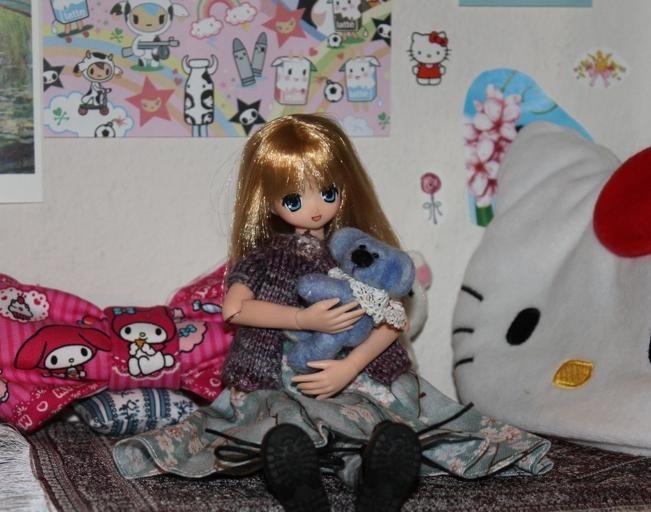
114;112;554;512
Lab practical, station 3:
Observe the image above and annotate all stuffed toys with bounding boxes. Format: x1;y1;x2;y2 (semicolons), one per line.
287;226;415;373
403;249;431;341
451;121;650;458
